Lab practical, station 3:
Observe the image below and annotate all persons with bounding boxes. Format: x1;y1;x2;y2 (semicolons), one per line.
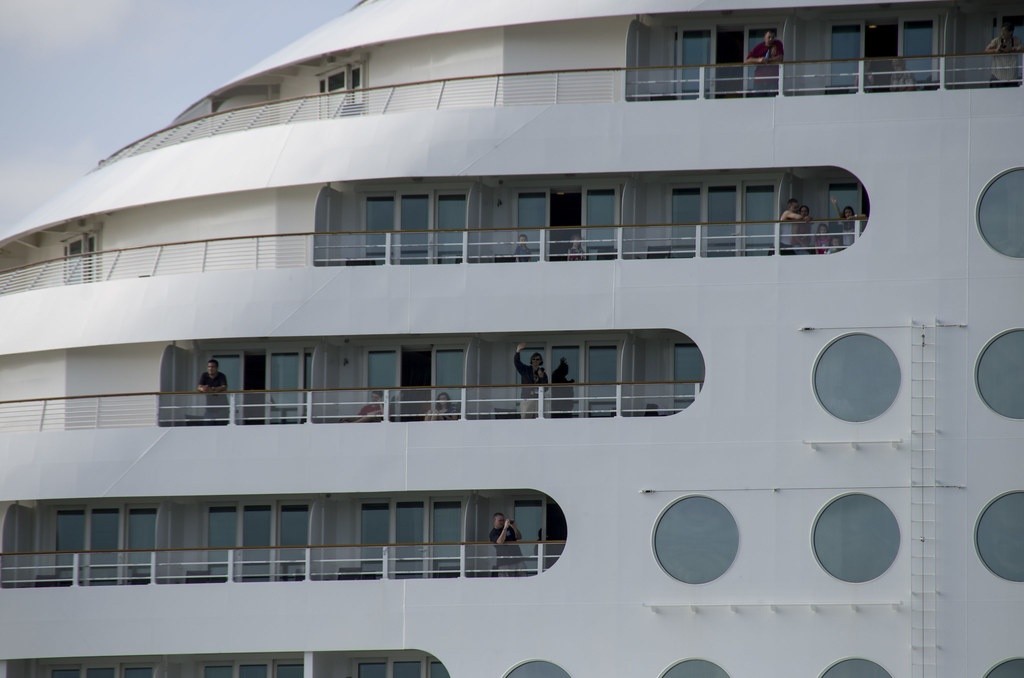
514;343;548;419
890;56;916;91
424;393;459;421
568;234;585;261
489;512;528;576
984;22;1024;88
854;57;881;92
515;234;531;262
198;359;229;425
780;196;867;255
354;390;384;423
745;30;784;97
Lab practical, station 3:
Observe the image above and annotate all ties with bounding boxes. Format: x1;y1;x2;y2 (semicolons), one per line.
765;48;770;59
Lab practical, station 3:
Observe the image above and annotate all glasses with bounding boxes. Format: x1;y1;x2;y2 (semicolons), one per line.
531;358;541;361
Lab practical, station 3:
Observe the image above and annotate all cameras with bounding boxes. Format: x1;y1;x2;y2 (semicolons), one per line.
509;520;513;524
